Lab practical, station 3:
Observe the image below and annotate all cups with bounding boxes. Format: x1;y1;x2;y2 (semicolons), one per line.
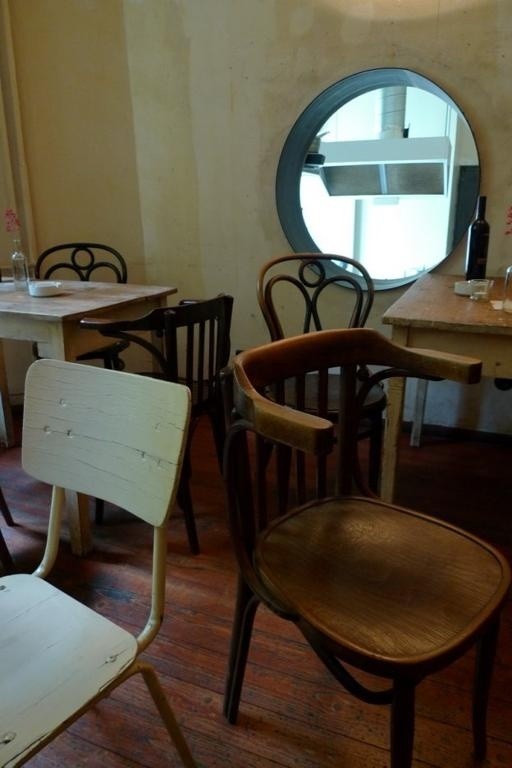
468;279;490;303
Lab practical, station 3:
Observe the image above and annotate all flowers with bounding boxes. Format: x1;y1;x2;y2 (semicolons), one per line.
3;204;20;241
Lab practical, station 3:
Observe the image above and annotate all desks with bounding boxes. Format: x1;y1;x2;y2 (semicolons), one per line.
381;273;511;505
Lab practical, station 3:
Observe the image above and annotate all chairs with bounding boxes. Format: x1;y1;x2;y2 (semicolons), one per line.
0;242;384;555
225;329;511;768
1;360;197;768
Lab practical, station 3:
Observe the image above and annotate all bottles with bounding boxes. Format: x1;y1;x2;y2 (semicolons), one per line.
503;266;512;315
11;239;30;292
464;196;491;282
